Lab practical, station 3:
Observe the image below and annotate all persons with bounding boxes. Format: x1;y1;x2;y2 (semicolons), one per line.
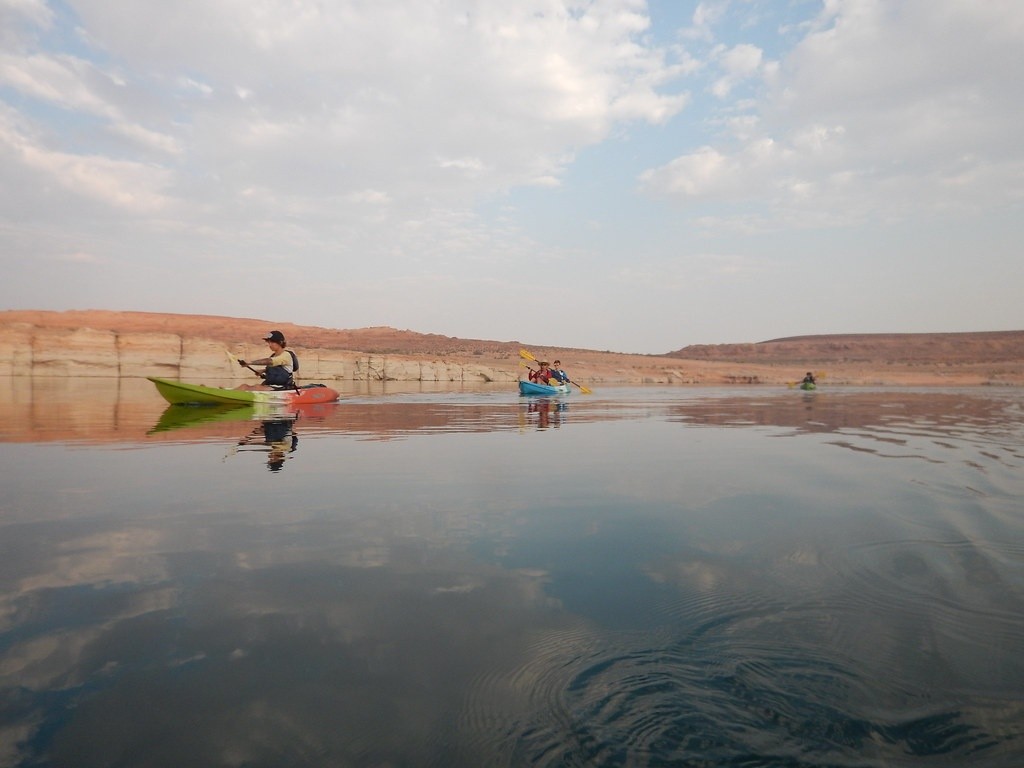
552;360;570;386
803;372;816;385
529;360;552;386
200;331;293;391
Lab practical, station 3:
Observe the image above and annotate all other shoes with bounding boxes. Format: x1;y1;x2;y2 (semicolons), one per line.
200;384;205;386
219;386;223;389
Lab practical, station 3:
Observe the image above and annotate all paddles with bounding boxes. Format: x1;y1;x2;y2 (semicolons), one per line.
225;349;266;379
519;361;559;386
517;347;592;394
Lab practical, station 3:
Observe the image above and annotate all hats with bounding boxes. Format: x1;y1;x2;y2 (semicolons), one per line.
537;357;551;366
262;331;287;348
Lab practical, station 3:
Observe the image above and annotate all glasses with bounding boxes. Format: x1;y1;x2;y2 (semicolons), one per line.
540;363;546;366
553;364;560;367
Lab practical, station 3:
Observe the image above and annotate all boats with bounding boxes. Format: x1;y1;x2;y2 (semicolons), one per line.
145;375;340;408
800;383;816;390
518;380;572;395
